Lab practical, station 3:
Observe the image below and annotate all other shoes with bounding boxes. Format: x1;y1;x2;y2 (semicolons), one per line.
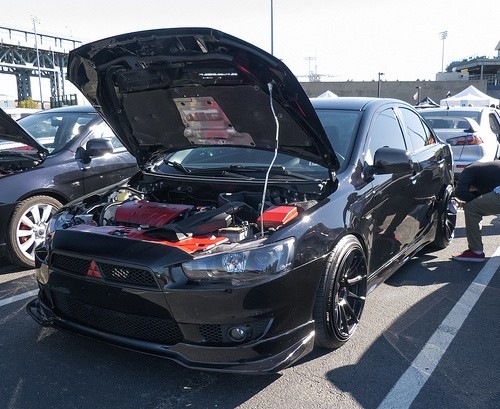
452;249;485;262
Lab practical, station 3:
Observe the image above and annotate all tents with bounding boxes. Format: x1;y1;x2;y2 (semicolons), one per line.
440;85;499;108
315;89;340;99
417;96;439;107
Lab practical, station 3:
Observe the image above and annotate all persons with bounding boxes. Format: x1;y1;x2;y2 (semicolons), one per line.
451;161;500;261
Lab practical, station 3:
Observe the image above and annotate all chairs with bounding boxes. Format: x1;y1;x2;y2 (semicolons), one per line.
432;120;450;128
77;125;84;132
457;120;468;127
323;124;339;148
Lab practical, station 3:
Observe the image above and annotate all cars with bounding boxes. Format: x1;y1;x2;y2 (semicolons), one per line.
23;26;456;375
420;106;499;171
1;100;134;266
